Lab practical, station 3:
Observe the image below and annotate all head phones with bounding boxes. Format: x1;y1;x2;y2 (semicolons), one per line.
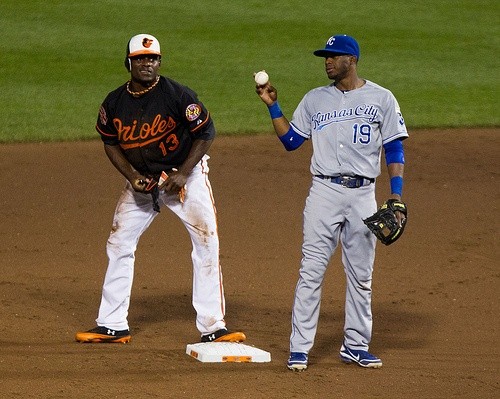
124;37;161;73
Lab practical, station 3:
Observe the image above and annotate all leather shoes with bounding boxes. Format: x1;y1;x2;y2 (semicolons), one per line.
318;175;375;188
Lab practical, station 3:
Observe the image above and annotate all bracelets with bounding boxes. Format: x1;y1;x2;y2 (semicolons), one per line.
268;102;283;119
390;176;403;196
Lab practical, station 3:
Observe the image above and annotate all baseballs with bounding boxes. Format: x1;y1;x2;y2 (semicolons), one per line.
254;71;268;86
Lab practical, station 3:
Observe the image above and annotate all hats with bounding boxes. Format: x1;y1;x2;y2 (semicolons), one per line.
127;33;161;57
313;34;360;59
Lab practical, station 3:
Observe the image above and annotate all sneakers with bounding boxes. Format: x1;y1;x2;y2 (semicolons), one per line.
200;329;247;343
339;339;383;368
286;352;308;372
75;326;131;344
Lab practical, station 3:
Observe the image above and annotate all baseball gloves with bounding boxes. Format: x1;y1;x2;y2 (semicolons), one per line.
359;200;408;245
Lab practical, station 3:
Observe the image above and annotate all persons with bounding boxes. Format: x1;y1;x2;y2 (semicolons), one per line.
253;35;409;371
76;34;246;344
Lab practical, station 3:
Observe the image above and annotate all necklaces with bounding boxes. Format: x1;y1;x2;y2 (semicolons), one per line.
127;77;159;95
